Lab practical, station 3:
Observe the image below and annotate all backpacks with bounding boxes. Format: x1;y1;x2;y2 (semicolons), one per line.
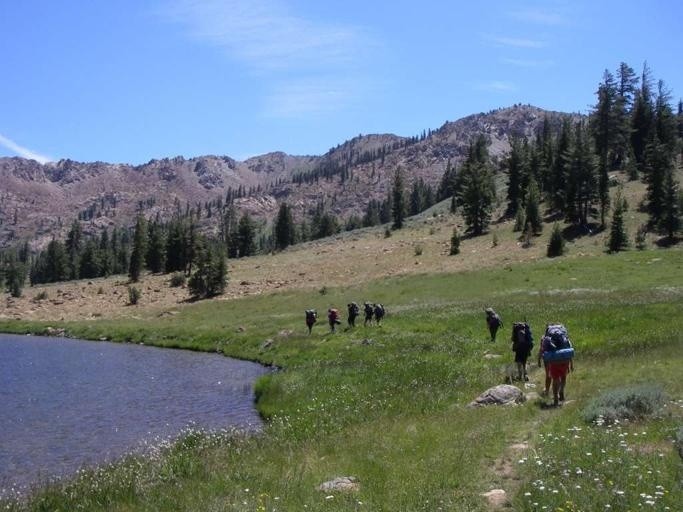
309;303;384;323
511;323;533;356
542;324;570;351
485;307;503;328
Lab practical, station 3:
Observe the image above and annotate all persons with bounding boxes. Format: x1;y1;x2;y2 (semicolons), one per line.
305;302;382;334
488;313;504;342
548;339;574;406
511;329;531;381
538;326;551;393
